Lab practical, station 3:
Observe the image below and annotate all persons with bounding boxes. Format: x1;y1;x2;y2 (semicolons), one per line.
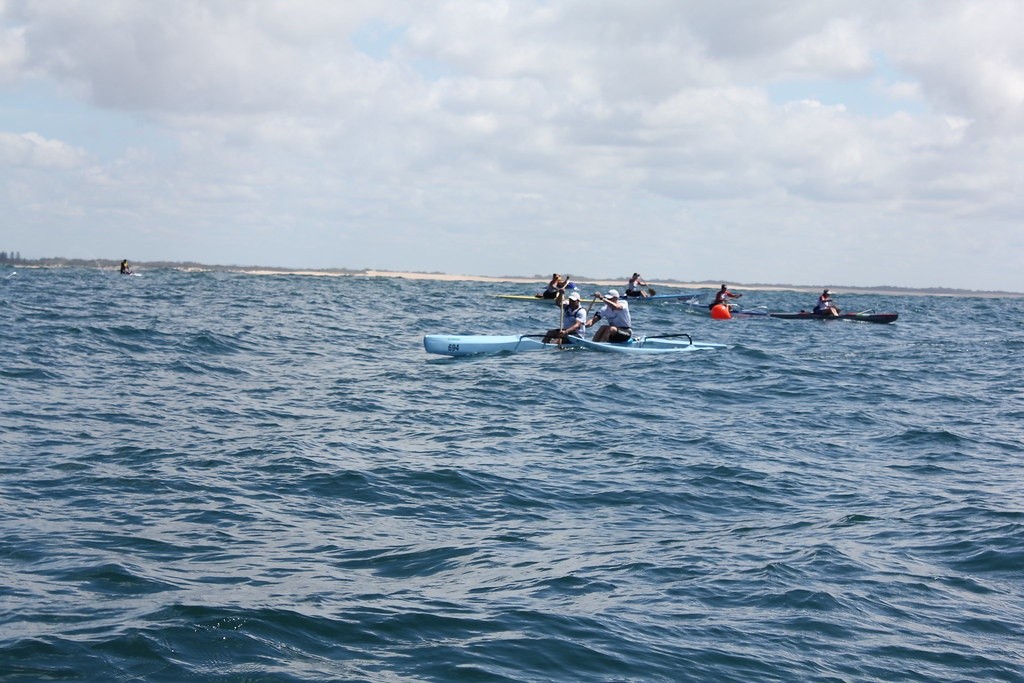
627;272;649;299
707;284;744;312
542;272;570;298
813;289;839;317
120;259;131;274
585;287;632;343
541;290;586;344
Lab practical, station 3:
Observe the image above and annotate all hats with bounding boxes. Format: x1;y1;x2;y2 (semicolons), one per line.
568;292;581;302
603;289;620;300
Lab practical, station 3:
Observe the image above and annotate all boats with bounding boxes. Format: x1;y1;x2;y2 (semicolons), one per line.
564;333;727;356
421;330;590;359
768;309;901;323
485;294;605;305
617;290;707;305
684;300;771;316
